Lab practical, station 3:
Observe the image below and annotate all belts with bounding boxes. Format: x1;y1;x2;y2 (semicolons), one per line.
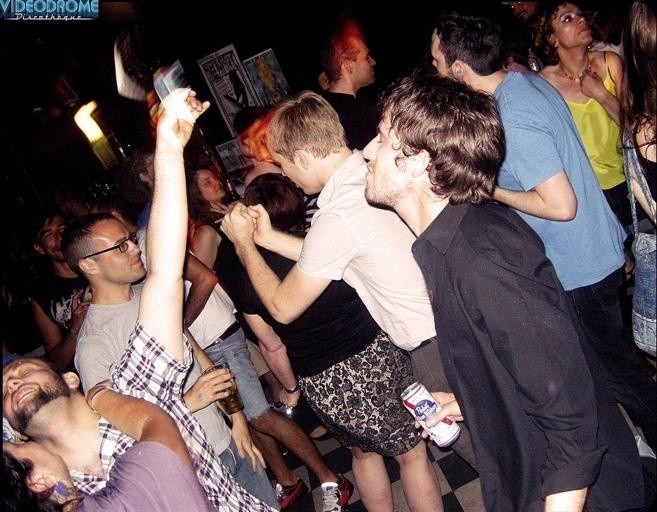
204;321;242;351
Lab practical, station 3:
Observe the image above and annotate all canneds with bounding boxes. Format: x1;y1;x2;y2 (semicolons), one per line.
399;381;461;449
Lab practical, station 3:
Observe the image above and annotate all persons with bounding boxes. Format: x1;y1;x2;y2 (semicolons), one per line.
0;1;657;512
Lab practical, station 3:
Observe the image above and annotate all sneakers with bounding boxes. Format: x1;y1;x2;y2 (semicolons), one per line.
272;405;294;414
319;472;353;512
273;476;310;511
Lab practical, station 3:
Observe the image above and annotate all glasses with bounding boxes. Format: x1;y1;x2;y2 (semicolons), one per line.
83;232;138;259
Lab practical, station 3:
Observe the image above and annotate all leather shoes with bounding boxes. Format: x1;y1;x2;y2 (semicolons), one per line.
307;429;333;442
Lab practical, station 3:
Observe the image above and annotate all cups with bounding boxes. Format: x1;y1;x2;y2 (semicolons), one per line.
201;362;244;415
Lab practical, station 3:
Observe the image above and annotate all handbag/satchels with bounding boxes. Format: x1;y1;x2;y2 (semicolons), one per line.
622;150;657;355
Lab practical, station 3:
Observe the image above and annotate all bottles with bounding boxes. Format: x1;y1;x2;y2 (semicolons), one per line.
223;178;241;202
526;49;540;73
